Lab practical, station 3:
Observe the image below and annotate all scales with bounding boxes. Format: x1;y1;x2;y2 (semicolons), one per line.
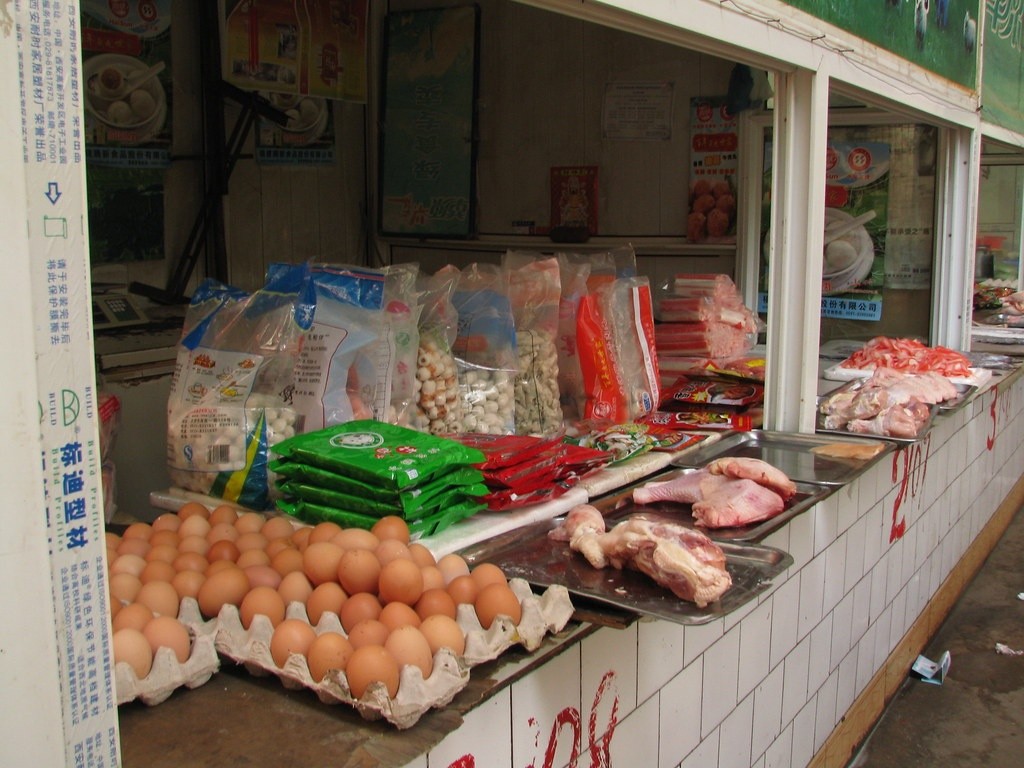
91;280;149;329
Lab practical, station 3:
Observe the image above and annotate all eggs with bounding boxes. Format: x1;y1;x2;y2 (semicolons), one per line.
103;501;524;702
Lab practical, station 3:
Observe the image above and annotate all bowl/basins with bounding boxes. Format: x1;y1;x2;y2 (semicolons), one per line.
276;97;325;134
764;207;867;281
84;53;162;130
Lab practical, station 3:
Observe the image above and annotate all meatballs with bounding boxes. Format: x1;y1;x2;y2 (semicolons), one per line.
384;325;563;434
687;181;734;239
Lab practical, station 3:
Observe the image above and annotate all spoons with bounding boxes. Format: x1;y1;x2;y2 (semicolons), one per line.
95;61;166;101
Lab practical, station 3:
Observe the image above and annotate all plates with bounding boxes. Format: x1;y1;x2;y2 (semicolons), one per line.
821;224;875;297
85;65;167;144
291;103;329;147
